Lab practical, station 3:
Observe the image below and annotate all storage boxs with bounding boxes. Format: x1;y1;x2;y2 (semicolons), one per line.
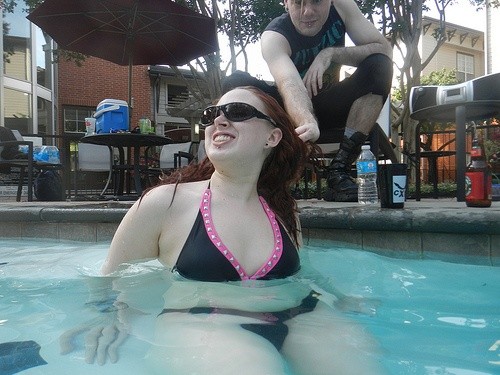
95;99;129;133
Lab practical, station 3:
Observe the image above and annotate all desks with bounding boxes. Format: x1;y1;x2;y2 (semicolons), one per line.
77;134;166;202
408;99;500;203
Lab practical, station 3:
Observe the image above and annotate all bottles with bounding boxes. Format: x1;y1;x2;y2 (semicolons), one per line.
356;145;378;205
465;132;492;209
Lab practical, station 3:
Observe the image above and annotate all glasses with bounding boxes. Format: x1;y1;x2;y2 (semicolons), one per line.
200;101;279;127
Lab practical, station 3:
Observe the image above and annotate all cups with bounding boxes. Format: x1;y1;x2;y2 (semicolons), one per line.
85;118;95;135
139;118;151;134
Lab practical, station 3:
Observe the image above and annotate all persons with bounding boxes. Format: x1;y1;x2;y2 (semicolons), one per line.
262;0;394;201
106;85;307;284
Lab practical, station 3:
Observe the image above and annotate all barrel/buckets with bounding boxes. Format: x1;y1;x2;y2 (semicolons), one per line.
377;164;408;209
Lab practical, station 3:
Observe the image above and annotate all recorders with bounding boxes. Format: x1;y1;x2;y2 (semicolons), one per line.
409;72;500;114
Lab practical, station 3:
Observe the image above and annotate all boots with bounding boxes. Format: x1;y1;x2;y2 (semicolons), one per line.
322;131;370;202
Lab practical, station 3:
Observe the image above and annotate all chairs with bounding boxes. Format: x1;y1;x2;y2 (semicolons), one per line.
0;126;60;202
141;141;197;188
407;128;477;200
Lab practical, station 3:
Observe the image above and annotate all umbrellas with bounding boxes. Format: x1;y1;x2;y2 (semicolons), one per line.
26;0;221;171
